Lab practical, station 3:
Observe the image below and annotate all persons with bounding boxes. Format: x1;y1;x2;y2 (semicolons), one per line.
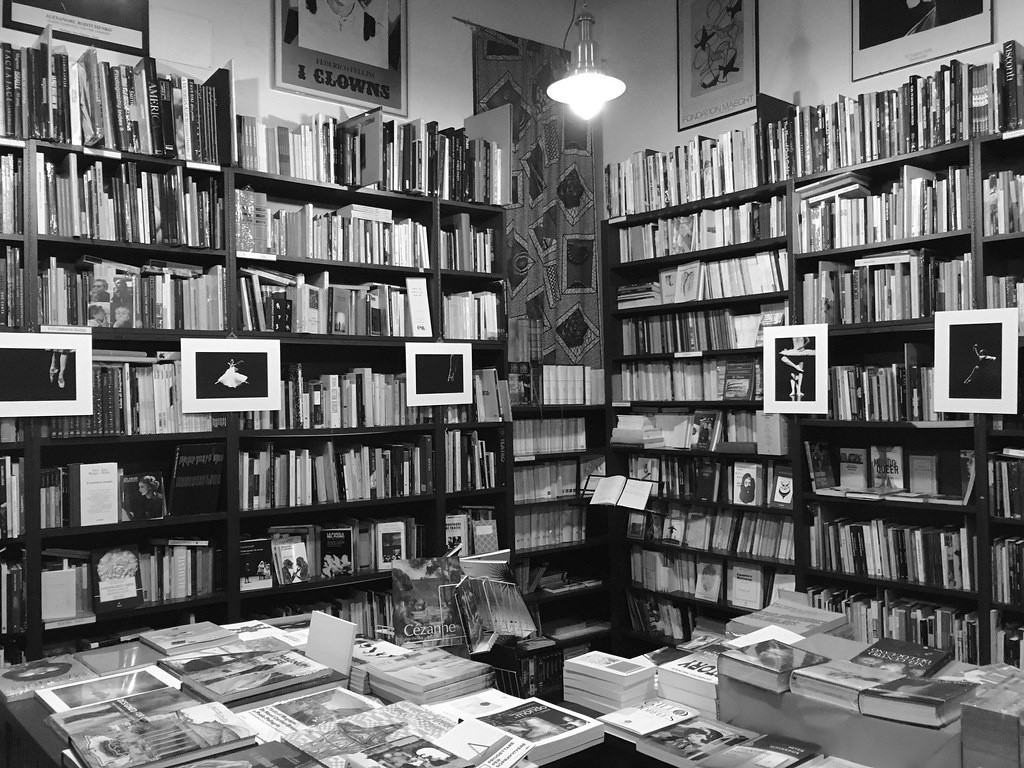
129;476;163;517
256;561;264;580
244;562;251;584
214;358;250;389
263;563;271;579
43;349;76;389
89;279;110;302
111;306;132;328
963;343;997;386
779;337;816;396
698;422;709;445
739;473;755;504
111;277;133;303
292;557;308;582
282;559;301;584
89;305;109;328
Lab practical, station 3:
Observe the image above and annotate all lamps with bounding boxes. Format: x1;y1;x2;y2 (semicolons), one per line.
545;0;627;121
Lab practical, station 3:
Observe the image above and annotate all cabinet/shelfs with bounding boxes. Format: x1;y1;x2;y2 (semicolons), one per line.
0;35;1024;669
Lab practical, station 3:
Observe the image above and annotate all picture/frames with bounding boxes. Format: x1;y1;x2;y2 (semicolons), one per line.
933;307;1018;414
0;333;96;416
274;0;410;120
180;336;282;415
763;322;829;414
1;0;151;57
403;341;473;407
674;0;759;134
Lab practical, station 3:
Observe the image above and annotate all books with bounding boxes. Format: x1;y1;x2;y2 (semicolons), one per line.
1;24;1024;768
